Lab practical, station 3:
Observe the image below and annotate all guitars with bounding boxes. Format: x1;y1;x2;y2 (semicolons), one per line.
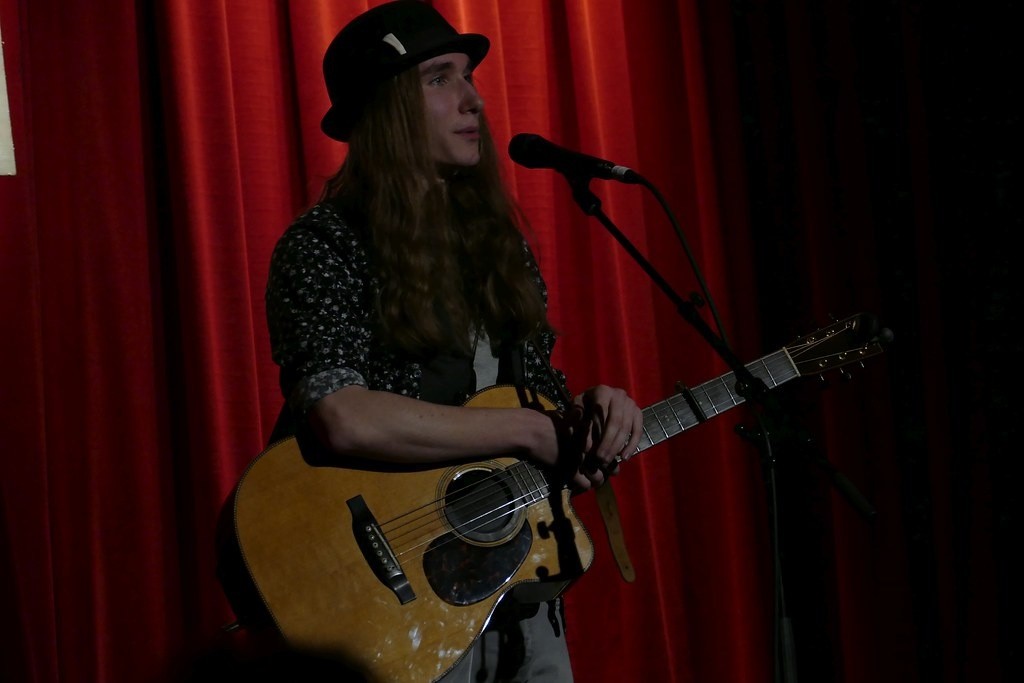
222;312;897;683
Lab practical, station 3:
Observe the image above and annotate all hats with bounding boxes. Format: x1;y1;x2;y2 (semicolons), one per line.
320;0;490;142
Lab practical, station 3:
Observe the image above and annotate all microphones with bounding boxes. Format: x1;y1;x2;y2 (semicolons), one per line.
507;132;634;183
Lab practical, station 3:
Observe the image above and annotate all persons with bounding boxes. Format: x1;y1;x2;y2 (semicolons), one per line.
265;0;642;683
